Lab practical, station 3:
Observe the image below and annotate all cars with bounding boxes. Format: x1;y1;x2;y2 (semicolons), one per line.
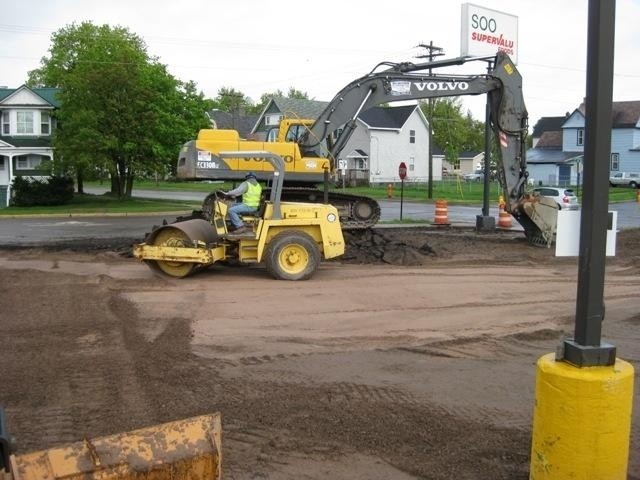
530;187;579;211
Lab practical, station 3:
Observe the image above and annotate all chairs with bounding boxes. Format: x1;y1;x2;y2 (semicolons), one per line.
239;197;266;224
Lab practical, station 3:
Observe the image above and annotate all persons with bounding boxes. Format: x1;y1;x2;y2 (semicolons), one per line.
217;172;263;234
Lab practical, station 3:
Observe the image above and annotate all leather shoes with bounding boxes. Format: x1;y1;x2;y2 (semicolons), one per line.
233;226;246;235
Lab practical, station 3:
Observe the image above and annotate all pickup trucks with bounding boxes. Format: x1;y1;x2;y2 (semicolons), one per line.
609;172;640;189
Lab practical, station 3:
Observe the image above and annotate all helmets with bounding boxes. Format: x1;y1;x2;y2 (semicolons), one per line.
245;172;256;178
